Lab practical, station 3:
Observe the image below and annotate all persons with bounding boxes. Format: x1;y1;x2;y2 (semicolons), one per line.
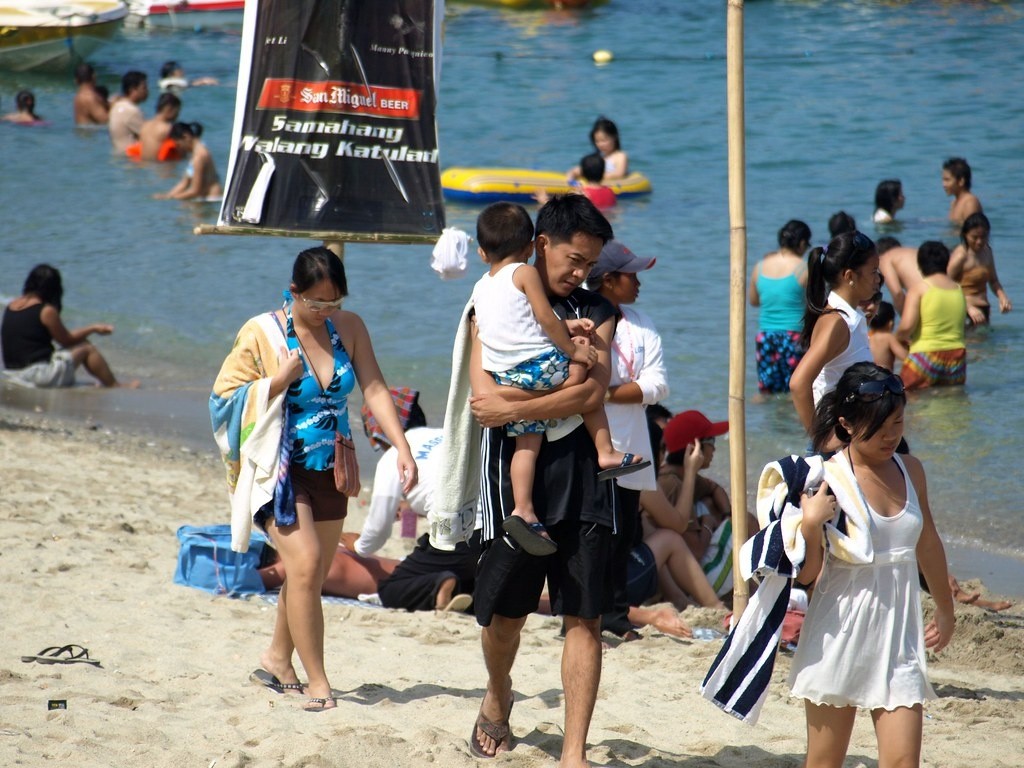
74;64;112;126
207;187;761;768
107;71;148;152
827;211;967;390
941;157;983;222
784;362;955;768
139;92;182;162
531;154;617;209
946;210;1013;327
748;218;811;393
567;118;628;181
168;121;222;200
872;180;906;223
161;61;222;87
788;212;880;461
0;90;41;123
0;263;140;390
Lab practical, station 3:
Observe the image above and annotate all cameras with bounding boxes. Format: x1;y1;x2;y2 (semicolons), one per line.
808;486;829;498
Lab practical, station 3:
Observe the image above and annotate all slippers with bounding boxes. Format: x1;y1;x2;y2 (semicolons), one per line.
502;516;558;556
251;669;304;696
597;453;651;481
303;691;338;711
470;690;514;758
21;644;100;666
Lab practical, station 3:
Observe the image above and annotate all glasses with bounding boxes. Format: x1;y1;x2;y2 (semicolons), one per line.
843;374;905;402
701;438;716;446
301;295;344;313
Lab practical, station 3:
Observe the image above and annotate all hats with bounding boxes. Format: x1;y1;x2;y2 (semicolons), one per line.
587;239;655;292
664;411;728;452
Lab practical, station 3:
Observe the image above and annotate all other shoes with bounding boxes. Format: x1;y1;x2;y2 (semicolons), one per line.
600;630;643;651
445;594;473;612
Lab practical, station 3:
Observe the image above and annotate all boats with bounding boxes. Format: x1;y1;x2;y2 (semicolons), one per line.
439;165;652;206
0;0;130;75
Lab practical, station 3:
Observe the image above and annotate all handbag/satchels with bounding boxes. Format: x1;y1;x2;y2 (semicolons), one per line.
724;607;804;653
334;431;360;497
703;456;810;721
